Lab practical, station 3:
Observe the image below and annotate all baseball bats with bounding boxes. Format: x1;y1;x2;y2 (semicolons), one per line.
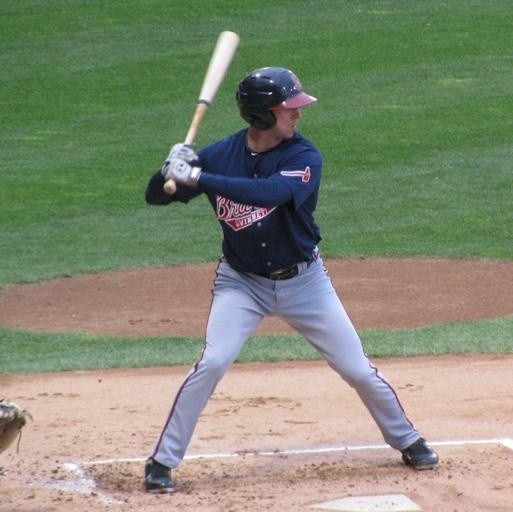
163;31;239;196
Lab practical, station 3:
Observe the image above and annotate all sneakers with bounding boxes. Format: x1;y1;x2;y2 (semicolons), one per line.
401;438;439;470
144;458;176;494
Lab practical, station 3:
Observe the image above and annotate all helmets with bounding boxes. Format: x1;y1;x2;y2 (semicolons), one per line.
236;66;318;130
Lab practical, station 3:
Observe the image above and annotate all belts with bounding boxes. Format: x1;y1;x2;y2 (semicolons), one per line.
255;259;315;281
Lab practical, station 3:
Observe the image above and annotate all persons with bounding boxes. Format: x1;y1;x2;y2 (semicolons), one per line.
143;66;437;494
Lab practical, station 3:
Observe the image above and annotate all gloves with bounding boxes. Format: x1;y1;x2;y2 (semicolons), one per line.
161;142;199;176
163;158;202;187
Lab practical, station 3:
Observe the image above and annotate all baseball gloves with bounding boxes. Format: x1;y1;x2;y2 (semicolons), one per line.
0;399;33;454
